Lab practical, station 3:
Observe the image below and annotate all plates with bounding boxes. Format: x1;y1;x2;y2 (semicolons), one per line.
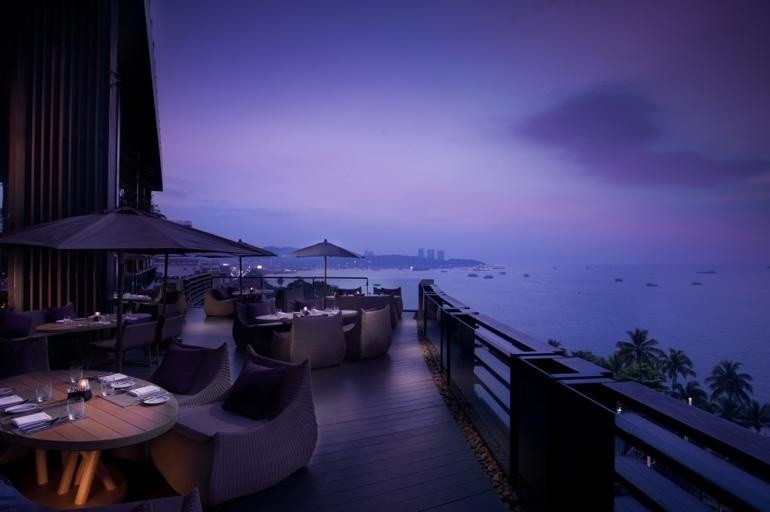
1;387;11;394
110;379;135;388
138;393;169;405
5;403;37;414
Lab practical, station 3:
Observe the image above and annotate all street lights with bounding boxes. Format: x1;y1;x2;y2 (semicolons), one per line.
257;264;264;290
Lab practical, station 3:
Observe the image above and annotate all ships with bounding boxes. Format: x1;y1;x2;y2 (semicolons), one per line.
409;264;430;272
470;263;490;272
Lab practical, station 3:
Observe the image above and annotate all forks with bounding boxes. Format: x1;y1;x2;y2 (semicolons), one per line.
21;416;60;430
1;398;29;409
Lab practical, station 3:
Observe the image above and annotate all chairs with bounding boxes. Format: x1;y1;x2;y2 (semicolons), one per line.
203;282;315;315
232;282;402;368
147;342;318;505
1;481;200;512
109;344;232;460
3;285;186;366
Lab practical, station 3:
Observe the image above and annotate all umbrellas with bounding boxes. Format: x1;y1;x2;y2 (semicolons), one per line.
233;237;277;294
286;238;365;293
0;196;249;375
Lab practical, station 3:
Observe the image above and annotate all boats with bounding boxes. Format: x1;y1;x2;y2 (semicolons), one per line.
692;281;701;285
467;271;532;280
696;270;717;273
492;267;505;269
441;269;448;273
616;278;624;282
647;283;658;287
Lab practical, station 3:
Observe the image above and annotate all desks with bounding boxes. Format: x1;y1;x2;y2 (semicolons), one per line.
110;292;151;314
36;313;151;366
234;287;273;302
255;308;357;322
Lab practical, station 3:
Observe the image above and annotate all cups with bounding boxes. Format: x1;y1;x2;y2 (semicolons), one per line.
36;381;53;402
98;310;132;326
100;376;116;397
272;306;340;318
64;315;71;327
69;366;84;383
66;397;85;420
